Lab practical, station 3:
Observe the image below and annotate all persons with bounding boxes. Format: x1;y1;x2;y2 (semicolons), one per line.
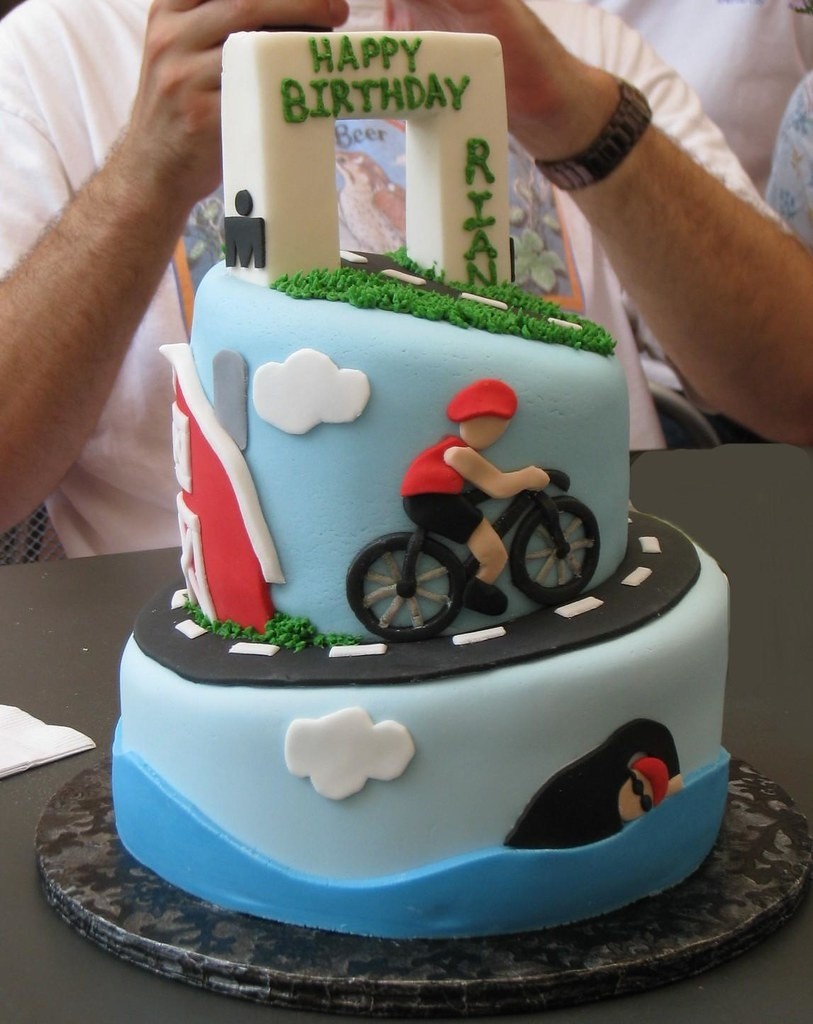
0;0;812;566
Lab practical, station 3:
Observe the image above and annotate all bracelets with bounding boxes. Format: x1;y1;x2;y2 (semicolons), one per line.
534;82;653;189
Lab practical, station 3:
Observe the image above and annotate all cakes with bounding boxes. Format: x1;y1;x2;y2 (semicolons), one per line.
110;29;730;940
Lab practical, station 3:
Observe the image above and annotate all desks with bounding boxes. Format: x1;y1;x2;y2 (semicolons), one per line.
0;443;813;1024
